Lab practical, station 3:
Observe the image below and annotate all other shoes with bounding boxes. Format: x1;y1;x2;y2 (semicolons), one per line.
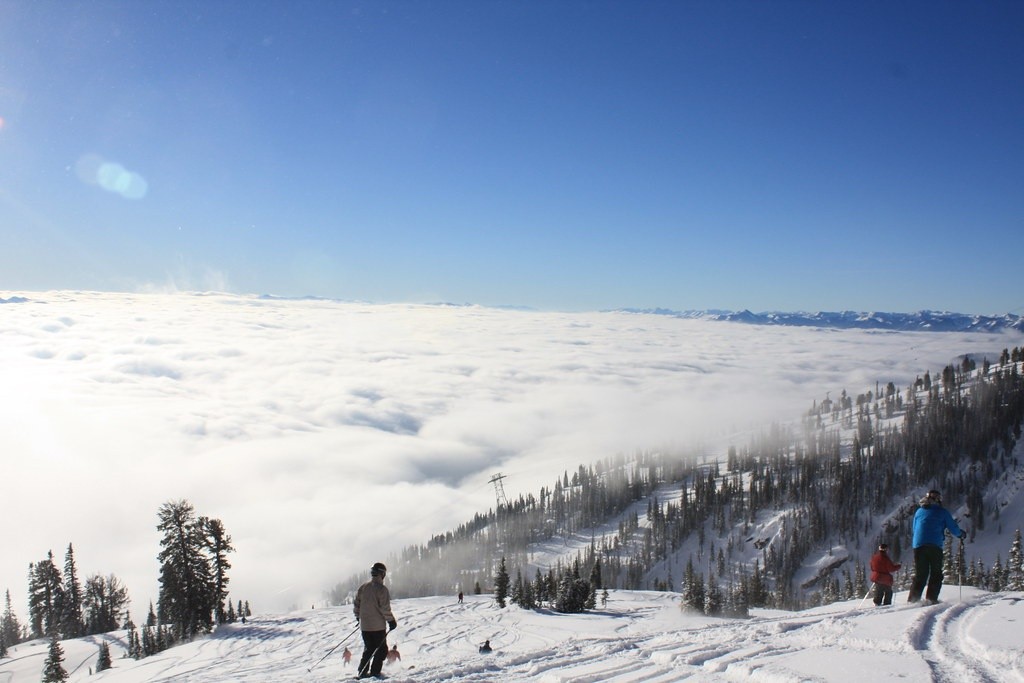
359;671;370;678
370;672;385;679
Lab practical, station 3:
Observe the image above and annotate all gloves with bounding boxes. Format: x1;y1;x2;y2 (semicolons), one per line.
356;617;359;622
958;528;967;539
388;619;397;631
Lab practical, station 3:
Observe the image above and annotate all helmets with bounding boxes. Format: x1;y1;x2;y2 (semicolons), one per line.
371;563;387;580
926;490;943;503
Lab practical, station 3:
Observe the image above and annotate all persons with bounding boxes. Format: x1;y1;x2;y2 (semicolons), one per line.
479;640;492;655
908;490;967;605
600;587;609;606
386;645;401;666
241;615;246;624
871;544;902;606
458;591;463;603
354;563;397;680
342;648;351;667
